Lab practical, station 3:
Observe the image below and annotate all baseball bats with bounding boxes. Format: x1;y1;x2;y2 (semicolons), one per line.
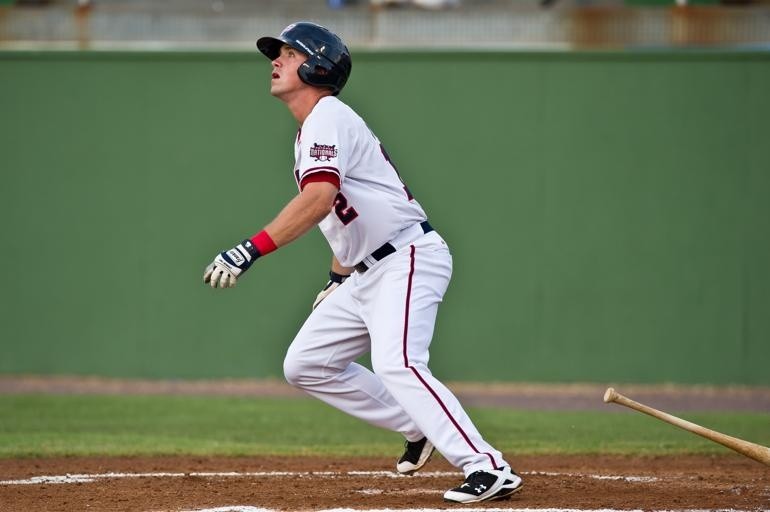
604;386;770;466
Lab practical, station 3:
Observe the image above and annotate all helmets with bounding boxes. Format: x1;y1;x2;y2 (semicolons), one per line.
256;20;352;96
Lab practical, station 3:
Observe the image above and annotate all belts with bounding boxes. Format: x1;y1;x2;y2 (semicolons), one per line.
354;222;435;275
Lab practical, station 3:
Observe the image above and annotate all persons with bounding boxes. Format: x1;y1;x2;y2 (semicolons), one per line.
203;21;523;504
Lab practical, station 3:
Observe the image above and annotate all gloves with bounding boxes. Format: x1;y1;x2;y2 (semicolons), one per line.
312;271;351;312
202;238;261;289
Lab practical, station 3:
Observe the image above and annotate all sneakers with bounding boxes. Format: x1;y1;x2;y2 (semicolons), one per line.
395;440;435;477
444;466;524;503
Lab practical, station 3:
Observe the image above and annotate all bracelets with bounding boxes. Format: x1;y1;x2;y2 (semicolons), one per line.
250;230;278;256
